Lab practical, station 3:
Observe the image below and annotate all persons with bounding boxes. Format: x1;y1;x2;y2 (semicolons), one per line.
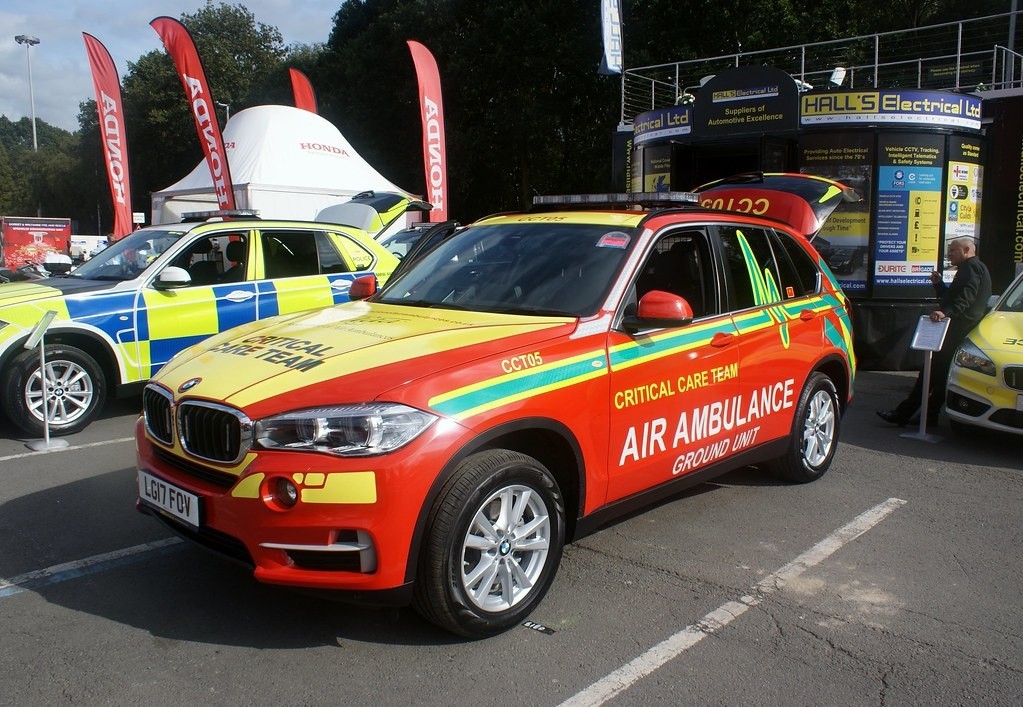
105;233;124;265
877;238;992;428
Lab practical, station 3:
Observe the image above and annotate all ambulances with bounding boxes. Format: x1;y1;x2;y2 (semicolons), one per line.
136;169;858;640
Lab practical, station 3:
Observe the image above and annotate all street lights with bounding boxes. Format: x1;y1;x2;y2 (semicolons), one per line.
214;100;230;123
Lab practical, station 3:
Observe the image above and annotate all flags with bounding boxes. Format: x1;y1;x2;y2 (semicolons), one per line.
597;1;623;75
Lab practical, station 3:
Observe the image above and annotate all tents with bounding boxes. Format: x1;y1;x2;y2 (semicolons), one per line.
152;104;422;225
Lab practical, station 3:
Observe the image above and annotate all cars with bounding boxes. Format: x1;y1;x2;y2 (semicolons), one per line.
1;190;436;439
938;268;1023;443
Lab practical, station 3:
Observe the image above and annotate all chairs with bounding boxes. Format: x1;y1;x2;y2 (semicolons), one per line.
637;241;701;316
547;240;584;279
188;239;218;287
222;241;246;282
